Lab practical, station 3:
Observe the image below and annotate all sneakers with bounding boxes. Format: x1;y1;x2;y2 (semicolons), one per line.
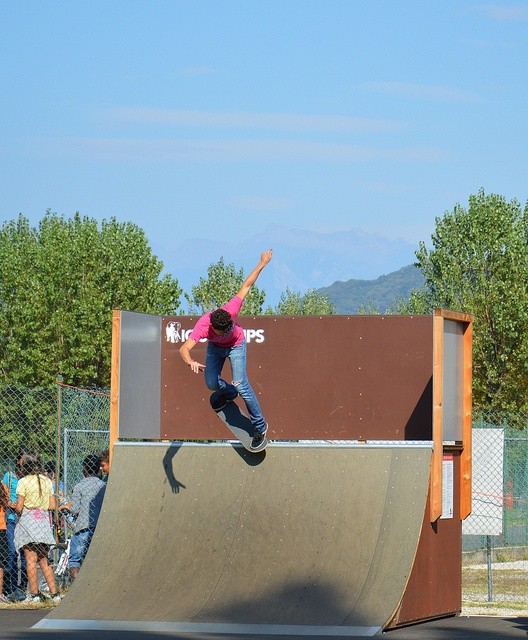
0;594;13;605
49;595;62;603
250;422;268;449
21;595;40;604
211;391;239;411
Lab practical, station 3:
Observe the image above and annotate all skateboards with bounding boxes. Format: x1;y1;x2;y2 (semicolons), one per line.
216;400;268;453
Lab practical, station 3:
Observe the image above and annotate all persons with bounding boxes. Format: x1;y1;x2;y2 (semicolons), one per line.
42;460;65;513
58;454;107;583
2;453;27;601
178;248;273;450
99;449;109;482
14;454;61;603
0;479;12;605
